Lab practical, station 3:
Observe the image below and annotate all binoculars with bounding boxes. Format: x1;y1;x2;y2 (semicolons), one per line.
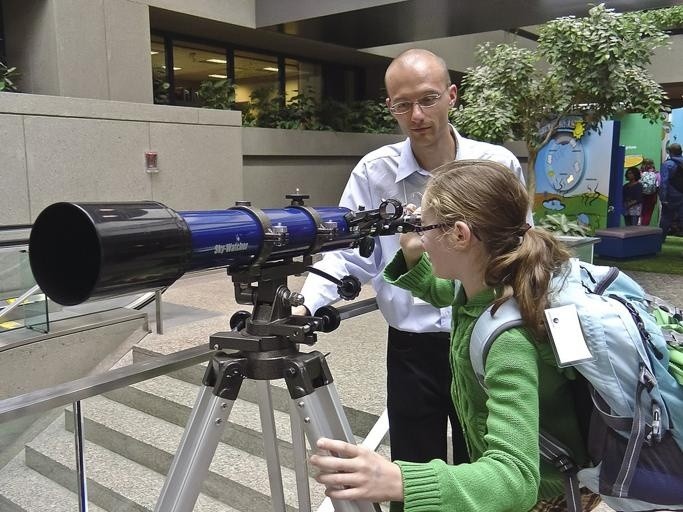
27;196;423;305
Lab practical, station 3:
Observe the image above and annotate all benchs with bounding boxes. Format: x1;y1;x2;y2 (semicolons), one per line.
593;224;663;262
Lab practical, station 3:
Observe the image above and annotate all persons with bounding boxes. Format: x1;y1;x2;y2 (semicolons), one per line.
310;158;603;512
286;47;536;511
638;159;661;226
656;142;683;243
622;166;641;227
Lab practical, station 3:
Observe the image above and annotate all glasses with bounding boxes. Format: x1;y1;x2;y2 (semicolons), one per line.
388;84;451;115
414;218;482;242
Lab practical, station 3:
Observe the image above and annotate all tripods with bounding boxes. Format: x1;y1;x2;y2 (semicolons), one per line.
152;328;382;511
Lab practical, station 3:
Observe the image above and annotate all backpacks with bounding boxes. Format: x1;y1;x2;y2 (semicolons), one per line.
640;169;659;195
664;158;683;193
468;257;683;512
